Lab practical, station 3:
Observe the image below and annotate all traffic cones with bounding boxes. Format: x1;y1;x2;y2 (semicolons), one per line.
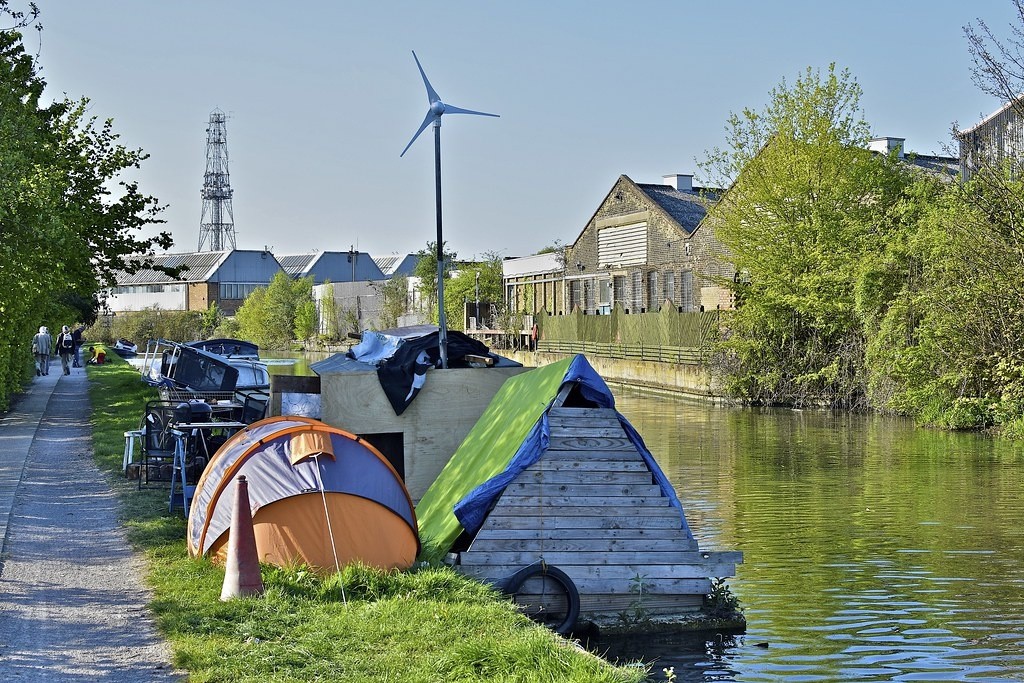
218;475;264;602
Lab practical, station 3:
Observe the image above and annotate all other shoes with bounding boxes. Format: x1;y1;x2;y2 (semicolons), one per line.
46;372;49;375
37;370;40;376
67;368;70;375
74;364;82;367
42;373;46;376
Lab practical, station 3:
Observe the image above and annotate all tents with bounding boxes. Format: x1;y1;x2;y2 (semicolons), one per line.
186;416;422;579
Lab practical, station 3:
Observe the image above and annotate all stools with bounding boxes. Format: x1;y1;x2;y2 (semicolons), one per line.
123;430;162;471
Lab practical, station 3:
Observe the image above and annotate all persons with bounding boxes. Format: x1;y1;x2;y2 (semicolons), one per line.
31;326;52;376
54;325;86;376
87;346;113;364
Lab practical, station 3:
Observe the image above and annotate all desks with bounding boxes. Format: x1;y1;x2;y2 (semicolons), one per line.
168;421;248;461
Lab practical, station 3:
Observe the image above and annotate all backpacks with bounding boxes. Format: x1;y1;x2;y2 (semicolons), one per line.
63;332;72;348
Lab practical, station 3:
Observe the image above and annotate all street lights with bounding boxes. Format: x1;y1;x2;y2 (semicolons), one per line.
476;272;481;324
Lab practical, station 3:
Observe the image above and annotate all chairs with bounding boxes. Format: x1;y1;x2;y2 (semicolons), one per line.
138;399;192;488
168;402;212;469
140;337;239;400
233;392;270;432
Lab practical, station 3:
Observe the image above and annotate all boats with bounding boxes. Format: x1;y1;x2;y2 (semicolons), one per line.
152;339;270;401
112;337;139;359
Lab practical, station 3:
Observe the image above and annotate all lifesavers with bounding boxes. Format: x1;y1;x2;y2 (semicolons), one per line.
504;563;579;638
